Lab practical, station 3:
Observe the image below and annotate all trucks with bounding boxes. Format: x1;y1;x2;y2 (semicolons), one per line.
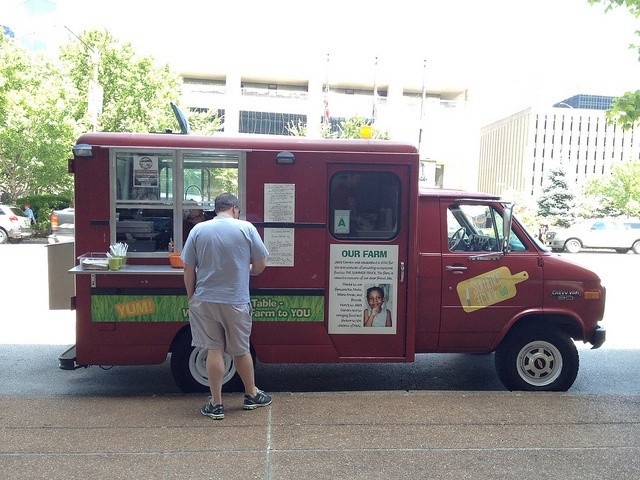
58;132;604;392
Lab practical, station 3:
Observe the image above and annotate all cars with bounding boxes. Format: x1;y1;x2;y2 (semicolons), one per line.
545;217;640;254
47;208;75;244
0;205;31;244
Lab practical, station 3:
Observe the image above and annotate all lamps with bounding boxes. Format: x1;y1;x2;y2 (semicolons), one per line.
275;150;296;166
72;144;93;159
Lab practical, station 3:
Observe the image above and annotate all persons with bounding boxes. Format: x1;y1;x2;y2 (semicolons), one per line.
365;288;392;327
168;200;212;249
334;173;375;232
180;194;273;420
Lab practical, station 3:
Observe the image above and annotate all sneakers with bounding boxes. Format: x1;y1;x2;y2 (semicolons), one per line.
200;395;225;420
242;387;273;410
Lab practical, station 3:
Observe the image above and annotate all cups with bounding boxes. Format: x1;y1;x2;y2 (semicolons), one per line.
108;258;122;270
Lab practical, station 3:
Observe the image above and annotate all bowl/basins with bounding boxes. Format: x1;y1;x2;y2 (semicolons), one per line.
170;255;184;268
